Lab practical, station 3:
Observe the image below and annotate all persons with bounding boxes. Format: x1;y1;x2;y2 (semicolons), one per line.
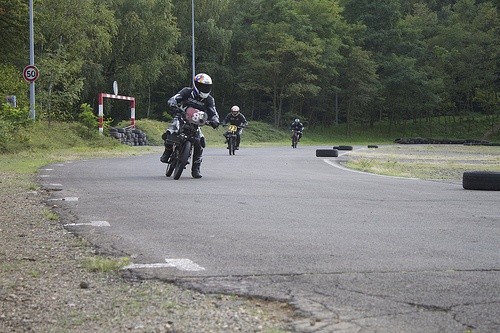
222;105;248;150
290;118;303;142
160;73;220;178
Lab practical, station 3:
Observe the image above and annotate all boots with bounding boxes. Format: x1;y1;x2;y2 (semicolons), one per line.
160;149;172;163
192;156;202;178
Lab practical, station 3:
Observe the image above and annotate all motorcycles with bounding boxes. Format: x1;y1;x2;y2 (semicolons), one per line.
291;128;302;148
164;96;224;180
222;124;245;156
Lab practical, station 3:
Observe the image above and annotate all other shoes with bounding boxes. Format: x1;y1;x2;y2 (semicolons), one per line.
236;144;239;150
227;146;230;149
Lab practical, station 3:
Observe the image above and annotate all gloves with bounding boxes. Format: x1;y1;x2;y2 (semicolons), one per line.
167;106;177;118
210;118;219;128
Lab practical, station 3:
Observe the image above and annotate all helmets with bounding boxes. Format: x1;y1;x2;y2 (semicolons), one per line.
231;106;239;117
194;73;212;98
295;119;299;122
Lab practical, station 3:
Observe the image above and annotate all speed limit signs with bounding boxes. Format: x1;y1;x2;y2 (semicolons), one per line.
23;65;40;82
